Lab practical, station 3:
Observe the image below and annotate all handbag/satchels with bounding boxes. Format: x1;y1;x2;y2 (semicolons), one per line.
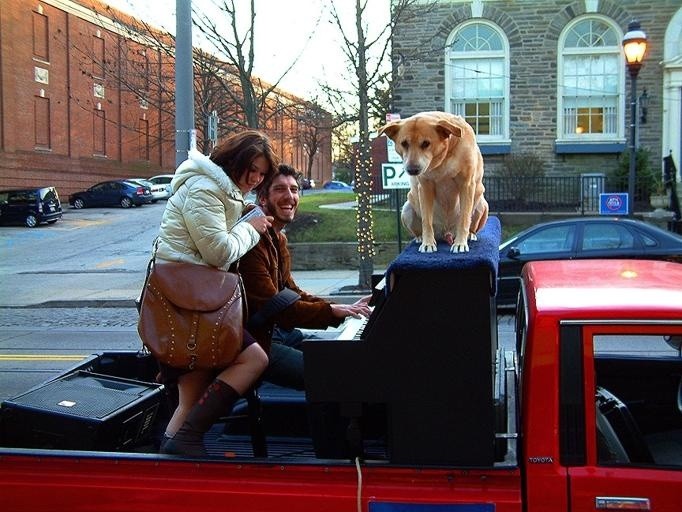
135;259;251;373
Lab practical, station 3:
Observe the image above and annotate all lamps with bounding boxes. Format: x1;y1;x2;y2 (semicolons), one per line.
638;87;650;123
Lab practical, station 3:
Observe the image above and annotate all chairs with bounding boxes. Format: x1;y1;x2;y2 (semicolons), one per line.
594;389;682;468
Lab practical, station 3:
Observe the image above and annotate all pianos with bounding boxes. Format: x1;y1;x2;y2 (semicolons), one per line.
300;212;506;465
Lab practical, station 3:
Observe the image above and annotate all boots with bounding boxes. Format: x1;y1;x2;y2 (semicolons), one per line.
162;376;242;457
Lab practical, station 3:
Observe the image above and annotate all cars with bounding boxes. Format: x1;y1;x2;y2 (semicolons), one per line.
68;179;153;209
0;186;62;228
496;216;682;315
324;181;354;191
147;174;174;200
122;178;169;203
301;178;315;189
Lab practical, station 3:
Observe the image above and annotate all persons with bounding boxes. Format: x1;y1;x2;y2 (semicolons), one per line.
151;129;278;456
226;164;372;394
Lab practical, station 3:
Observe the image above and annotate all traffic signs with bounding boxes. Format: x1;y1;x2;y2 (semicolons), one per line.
386;113;404;163
381;163;411;190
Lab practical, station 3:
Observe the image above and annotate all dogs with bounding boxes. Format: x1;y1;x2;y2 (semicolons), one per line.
374;109;490;256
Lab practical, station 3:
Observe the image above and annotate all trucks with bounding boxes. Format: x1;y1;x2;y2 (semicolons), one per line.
0;258;681;508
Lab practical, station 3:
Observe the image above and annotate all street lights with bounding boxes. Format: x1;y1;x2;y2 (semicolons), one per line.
622;20;648;218
